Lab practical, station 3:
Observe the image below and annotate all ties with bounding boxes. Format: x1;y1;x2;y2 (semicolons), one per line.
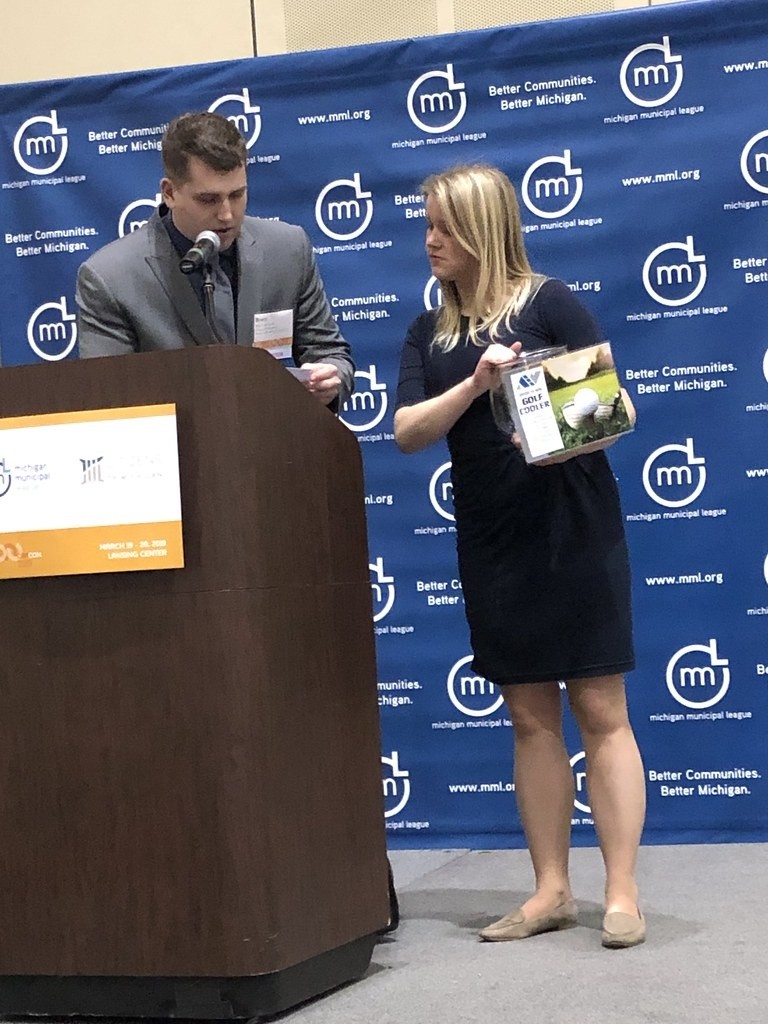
203;253;239;346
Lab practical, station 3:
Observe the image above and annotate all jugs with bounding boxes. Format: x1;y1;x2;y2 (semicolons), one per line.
490;345;569;464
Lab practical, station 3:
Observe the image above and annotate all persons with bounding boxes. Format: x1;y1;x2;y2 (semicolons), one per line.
74;113;356;418
395;163;649;949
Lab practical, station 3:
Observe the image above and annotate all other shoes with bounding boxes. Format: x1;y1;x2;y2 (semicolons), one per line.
601;903;645;948
475;898;581;944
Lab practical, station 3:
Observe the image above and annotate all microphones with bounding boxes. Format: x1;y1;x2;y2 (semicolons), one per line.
179;229;221;275
